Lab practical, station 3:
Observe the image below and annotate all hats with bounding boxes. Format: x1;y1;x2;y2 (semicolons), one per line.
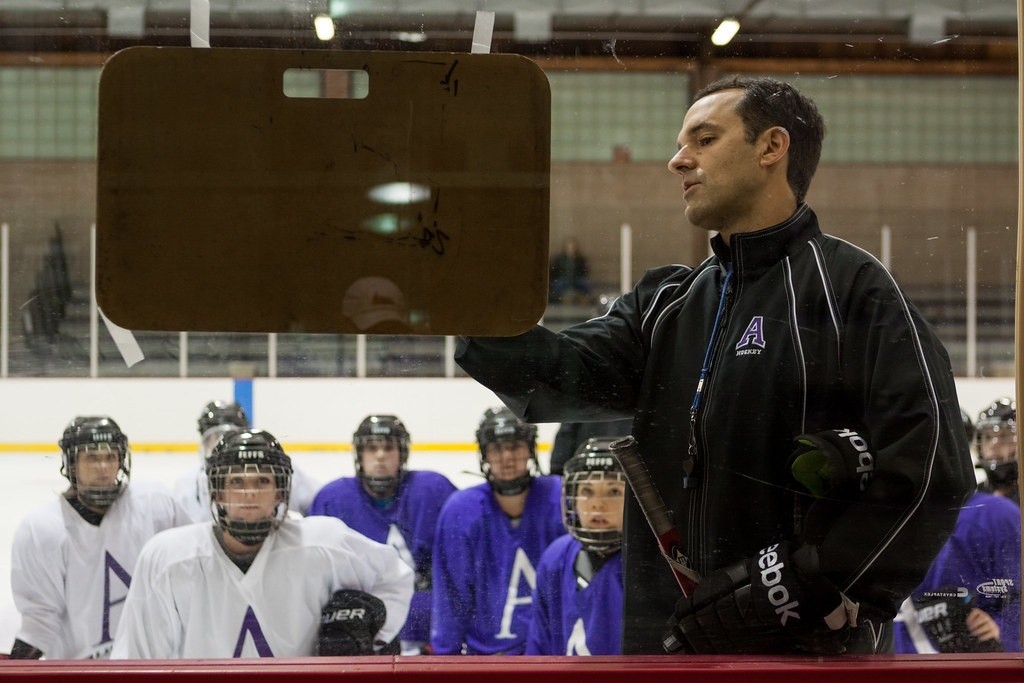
342;275;409;331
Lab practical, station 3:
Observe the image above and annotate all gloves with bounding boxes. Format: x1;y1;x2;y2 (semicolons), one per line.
662;541;847;657
317;588;385;656
786;424;874;541
913;587;1001;653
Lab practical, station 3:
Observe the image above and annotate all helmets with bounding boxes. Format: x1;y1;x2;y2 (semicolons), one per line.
198;400;292;540
476;404;539;496
560;436;630;559
959;398;1016;485
355;413;411;500
61;418;130;511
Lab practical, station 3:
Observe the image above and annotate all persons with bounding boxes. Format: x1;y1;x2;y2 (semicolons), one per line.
456;75;977;655
7;386;1023;660
548;238;602;307
